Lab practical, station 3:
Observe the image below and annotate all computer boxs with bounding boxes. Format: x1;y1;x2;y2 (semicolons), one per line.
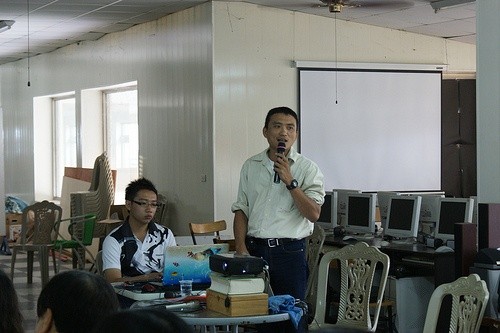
383;271;436;333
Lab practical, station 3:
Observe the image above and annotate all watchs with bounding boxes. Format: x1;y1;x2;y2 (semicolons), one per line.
286;179;298;190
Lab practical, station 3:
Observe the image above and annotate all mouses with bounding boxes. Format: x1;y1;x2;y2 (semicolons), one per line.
342;236;353;240
380;241;390;247
436;245;455;252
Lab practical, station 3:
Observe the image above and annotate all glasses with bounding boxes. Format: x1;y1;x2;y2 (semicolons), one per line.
129;200;161;208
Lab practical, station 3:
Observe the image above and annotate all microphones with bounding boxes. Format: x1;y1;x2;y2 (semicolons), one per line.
162;299;201;311
274;142;286;184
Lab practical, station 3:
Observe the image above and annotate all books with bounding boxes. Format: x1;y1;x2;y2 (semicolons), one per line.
209;272;266;295
114;285;165;301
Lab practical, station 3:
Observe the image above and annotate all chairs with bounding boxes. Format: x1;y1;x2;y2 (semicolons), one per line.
11;200;62;287
51;213;97;273
307;242;390;333
423;274;490;333
190;220;226;245
304;225;326;300
70;153;169;274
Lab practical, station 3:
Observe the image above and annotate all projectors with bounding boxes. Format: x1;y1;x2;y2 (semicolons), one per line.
209;253;267;276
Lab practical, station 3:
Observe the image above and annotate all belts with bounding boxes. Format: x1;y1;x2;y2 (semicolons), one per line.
246;238;305;248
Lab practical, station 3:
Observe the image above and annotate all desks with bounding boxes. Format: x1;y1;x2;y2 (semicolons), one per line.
309;228;456;281
122;298;307;333
108;279;207;301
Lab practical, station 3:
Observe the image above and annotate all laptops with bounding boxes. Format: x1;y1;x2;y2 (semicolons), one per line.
147;242;229;290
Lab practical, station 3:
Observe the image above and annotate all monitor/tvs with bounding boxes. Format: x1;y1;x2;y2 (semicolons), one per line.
315;189;474;240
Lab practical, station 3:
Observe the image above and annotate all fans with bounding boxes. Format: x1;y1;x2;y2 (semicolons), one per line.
310;0;364;105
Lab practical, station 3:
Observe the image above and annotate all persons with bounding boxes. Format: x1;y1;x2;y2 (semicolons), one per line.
0;268;25;333
232;106;327;333
101;177;177;283
35;270;120;333
92;306;194;333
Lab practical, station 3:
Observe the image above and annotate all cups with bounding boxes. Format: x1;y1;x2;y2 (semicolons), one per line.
178;279;194;298
